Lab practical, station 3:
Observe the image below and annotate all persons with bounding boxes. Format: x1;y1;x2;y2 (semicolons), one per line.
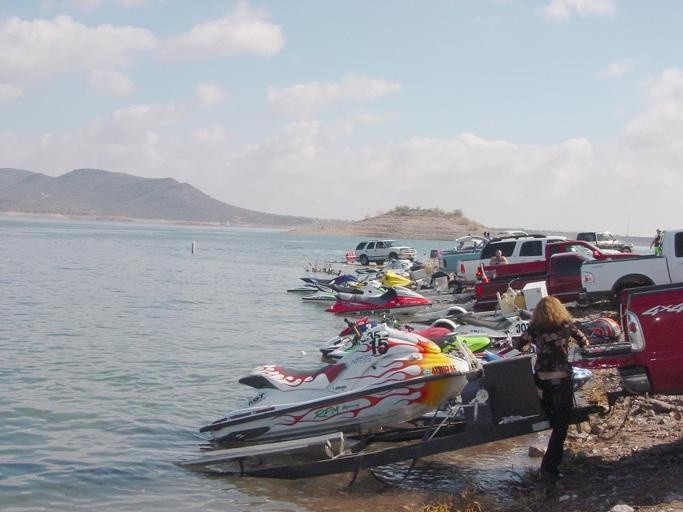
649;228;660;254
489;249;507;265
658;230;664;254
515;294;589;478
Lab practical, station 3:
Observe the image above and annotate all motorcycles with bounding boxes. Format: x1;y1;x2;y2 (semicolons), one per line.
289;265;431;313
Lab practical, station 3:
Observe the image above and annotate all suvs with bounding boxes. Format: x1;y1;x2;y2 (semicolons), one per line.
354;240;416;264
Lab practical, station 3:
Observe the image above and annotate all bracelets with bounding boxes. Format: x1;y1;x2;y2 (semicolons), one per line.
495;262;499;264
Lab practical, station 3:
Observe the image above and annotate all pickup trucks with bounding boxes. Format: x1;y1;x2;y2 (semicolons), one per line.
440;229;619;283
475;238;641;310
572;283;683;396
580;228;683;301
